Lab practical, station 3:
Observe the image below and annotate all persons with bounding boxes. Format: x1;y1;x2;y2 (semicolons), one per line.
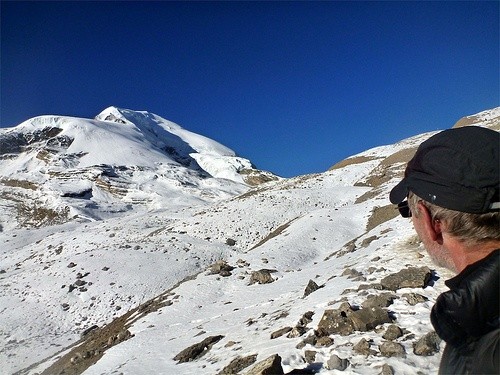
389;125;500;375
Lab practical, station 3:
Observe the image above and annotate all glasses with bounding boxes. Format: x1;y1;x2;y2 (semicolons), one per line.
398;199;440;223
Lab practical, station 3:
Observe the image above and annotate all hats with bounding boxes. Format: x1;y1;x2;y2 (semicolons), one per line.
388;125;500;214
